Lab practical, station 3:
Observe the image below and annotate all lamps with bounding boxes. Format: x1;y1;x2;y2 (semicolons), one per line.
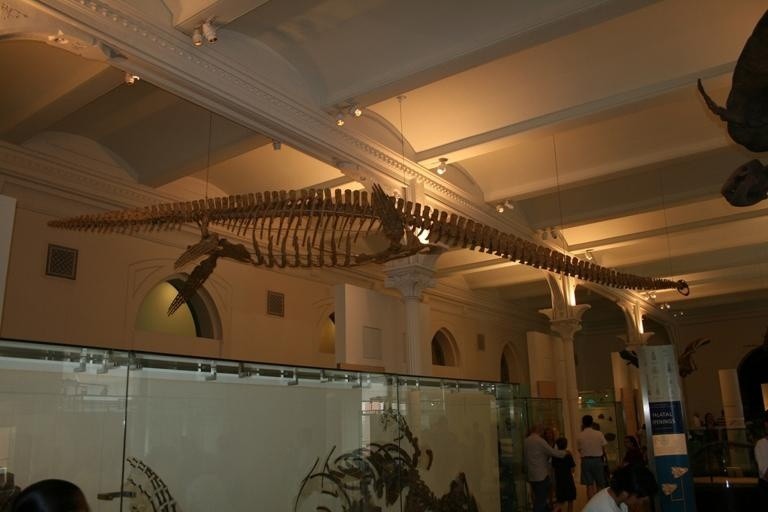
336;102;365;127
496;201;514;215
193;14;220;45
542;225;558;241
583;250;593;260
436;158;448;175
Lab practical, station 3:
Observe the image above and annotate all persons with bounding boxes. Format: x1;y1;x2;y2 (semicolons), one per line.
9;477;88;510
498;408;767;511
